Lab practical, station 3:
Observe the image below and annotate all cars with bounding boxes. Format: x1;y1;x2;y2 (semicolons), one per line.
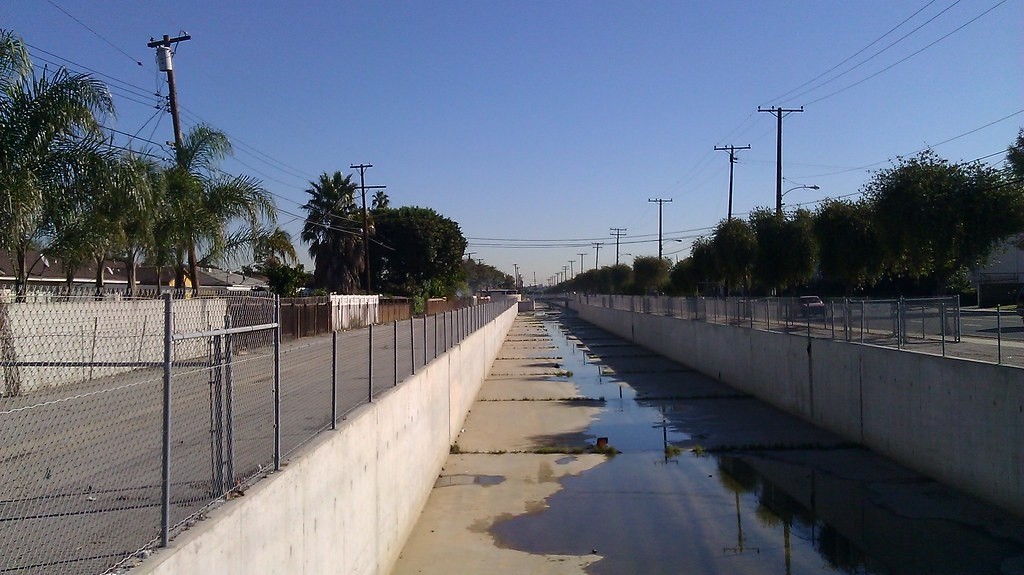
790;296;827;318
680;295;706;312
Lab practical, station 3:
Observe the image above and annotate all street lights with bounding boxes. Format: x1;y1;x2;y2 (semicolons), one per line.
617;253;631;266
659;239;682;260
776;186;820;213
